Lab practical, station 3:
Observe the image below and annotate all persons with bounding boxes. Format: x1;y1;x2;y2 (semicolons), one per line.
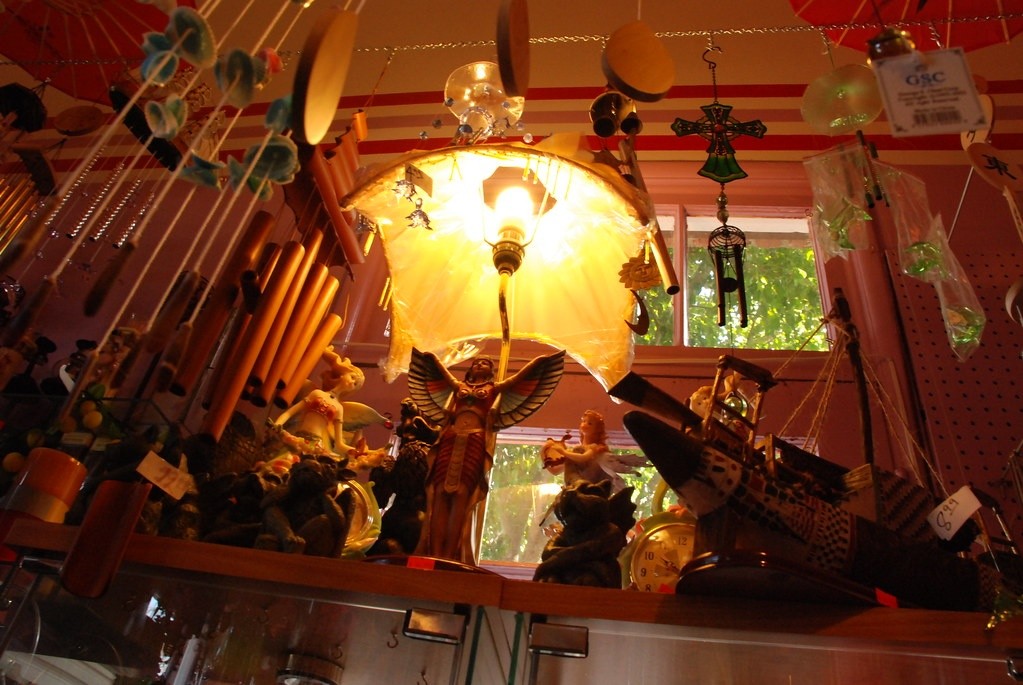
272;344;365;486
690;370;745;435
542;408;614;502
423;352;550;561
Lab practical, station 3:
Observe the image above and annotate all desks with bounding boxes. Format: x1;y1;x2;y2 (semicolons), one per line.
5;519;1023;685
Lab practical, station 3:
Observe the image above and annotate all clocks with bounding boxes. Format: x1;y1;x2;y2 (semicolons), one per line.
617;478;698;592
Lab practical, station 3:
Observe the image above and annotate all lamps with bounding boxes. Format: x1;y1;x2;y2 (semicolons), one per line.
479;163;555;382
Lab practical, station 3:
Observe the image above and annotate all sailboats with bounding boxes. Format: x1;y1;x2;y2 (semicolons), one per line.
605;287;980;608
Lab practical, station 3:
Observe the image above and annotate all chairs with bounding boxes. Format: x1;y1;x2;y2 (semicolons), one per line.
0;480;153;685
